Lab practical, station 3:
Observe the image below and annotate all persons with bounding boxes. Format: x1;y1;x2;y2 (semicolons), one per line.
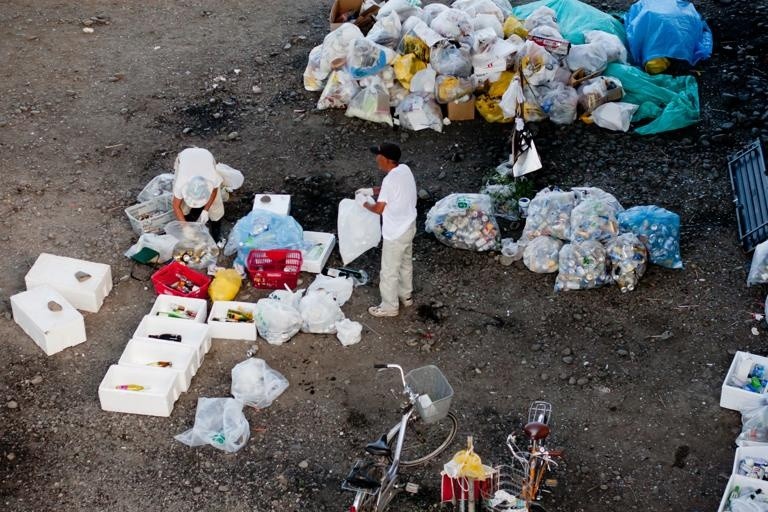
354;139;419;318
172;145;225;242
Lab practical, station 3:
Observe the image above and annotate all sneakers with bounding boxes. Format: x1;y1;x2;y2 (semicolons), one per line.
399;296;414;307
367;305;399;318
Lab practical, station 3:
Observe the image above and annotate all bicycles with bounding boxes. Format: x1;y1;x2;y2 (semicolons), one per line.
341;364;458;512
478;401;562;512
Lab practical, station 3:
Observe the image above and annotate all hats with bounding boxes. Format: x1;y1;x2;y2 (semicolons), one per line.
181;176;213;209
369;142;402;162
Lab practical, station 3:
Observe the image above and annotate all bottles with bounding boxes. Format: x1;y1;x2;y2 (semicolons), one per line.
146;361;172;369
323;266;362;282
728;486;740;502
115;384;152;392
156;311;189;319
148;334;182;343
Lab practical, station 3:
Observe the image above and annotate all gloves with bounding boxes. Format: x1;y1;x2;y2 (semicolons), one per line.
356;194;369;208
355;187;375;197
196;210;210;225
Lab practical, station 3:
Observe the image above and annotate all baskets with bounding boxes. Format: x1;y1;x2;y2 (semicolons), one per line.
151;261;209;304
247;248;303;291
